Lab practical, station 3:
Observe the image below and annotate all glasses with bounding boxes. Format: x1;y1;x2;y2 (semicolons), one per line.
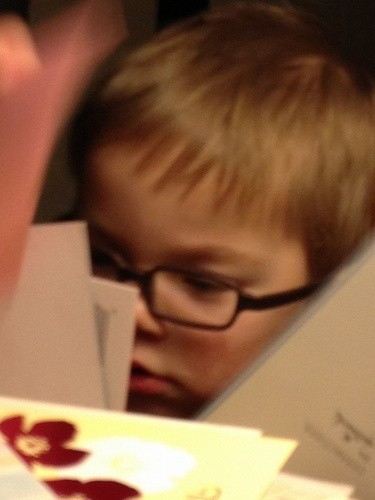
54;211;321;331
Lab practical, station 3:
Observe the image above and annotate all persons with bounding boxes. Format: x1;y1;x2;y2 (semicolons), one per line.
0;0;125;353
67;1;375;422
